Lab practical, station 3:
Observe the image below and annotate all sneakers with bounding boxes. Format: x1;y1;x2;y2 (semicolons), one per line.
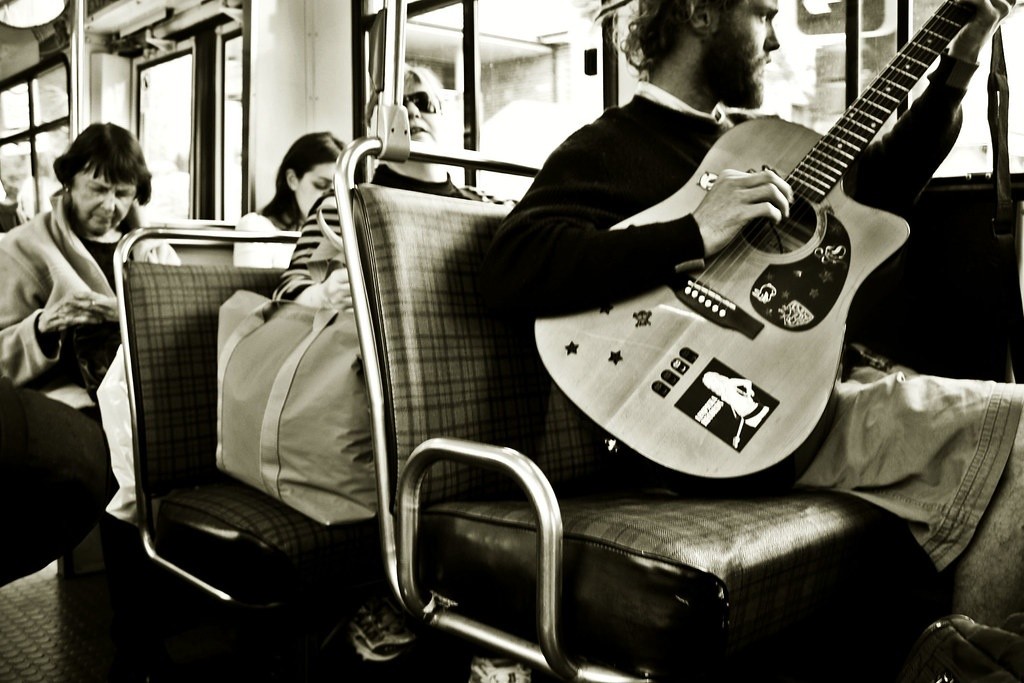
468;656;532;683
346;598;417;662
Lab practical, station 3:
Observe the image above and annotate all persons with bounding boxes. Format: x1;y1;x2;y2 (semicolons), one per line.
0;66;520;683
470;0;1024;625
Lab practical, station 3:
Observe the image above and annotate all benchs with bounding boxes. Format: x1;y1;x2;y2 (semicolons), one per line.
336;138;864;683
104;231;344;601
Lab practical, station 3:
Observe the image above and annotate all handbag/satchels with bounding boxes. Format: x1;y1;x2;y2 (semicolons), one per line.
215;289;379;526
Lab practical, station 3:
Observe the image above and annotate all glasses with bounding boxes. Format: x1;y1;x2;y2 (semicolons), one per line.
403;91;436;113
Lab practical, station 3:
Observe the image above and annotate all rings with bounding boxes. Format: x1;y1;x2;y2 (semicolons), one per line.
89;300;97;310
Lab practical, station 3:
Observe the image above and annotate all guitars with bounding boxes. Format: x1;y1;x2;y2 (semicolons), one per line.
533;0;982;481
